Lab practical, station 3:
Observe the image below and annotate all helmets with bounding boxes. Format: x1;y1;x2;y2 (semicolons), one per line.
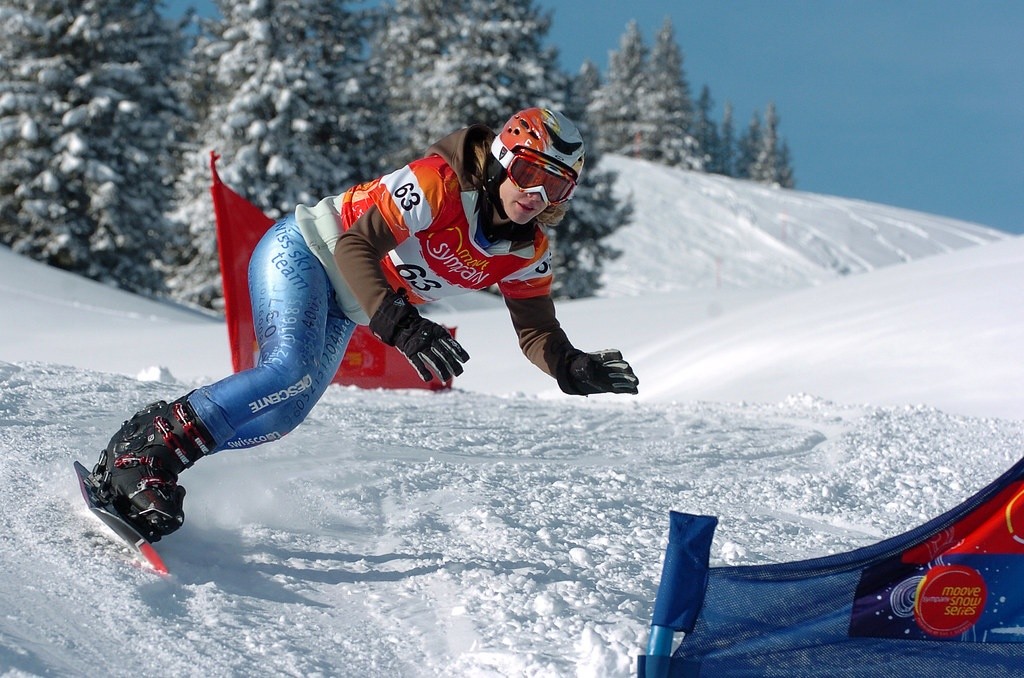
485;107;585;207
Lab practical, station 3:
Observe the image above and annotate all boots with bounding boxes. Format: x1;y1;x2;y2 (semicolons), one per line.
98;387;217;536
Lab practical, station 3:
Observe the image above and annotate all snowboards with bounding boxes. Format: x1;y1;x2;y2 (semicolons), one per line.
73;459;171;578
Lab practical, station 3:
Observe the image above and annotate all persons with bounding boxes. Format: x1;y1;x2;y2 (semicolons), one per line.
101;107;639;539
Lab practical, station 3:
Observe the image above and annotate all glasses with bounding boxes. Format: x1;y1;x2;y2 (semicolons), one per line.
507;154;578;207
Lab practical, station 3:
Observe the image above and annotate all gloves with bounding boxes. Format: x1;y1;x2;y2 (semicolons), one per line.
368;292;471;383
555;347;639;396
